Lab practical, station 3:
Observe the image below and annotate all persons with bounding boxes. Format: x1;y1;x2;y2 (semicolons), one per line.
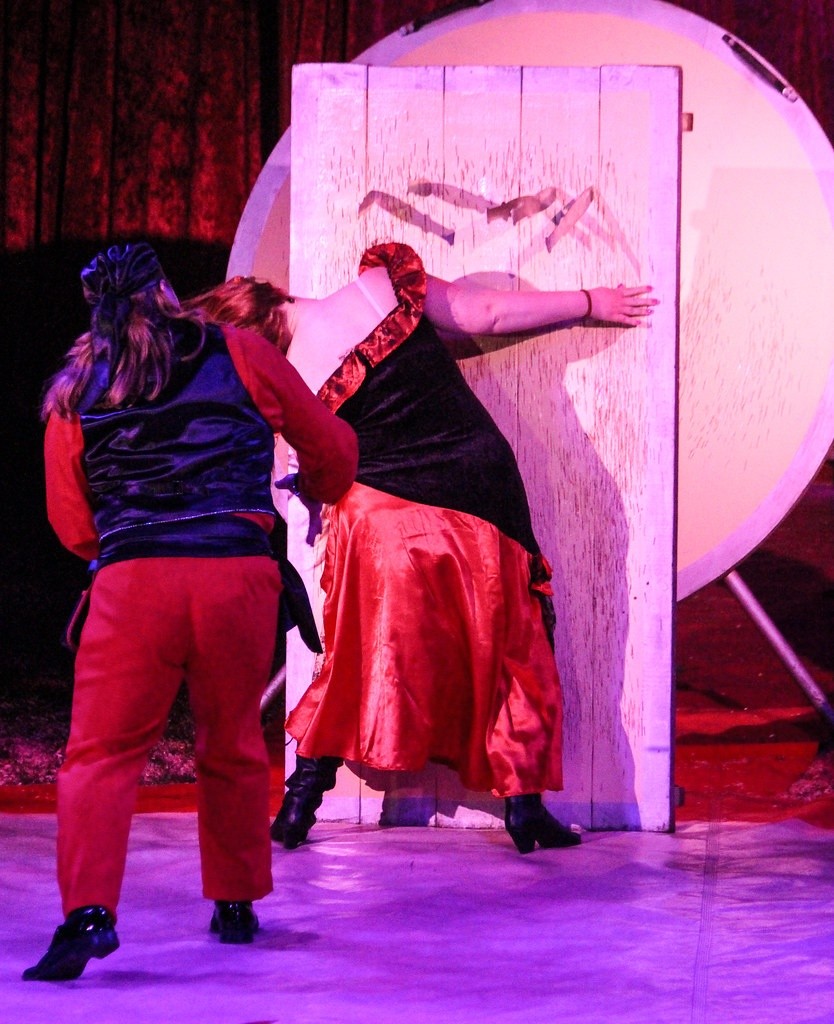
23;242;360;982
180;245;660;852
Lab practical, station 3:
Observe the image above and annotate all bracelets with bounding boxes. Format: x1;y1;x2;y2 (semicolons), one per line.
580;289;592;318
293;474;300;496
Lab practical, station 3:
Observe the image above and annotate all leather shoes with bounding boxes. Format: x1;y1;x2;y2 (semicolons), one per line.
208;897;259;946
21;904;120;982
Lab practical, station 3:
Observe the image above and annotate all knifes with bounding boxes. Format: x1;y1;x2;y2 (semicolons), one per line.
406;183;433;195
510;187;556;225
545;188;595;253
358;189;377;213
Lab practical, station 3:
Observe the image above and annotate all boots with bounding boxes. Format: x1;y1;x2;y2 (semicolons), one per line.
269;739;345;850
504;792;582;855
